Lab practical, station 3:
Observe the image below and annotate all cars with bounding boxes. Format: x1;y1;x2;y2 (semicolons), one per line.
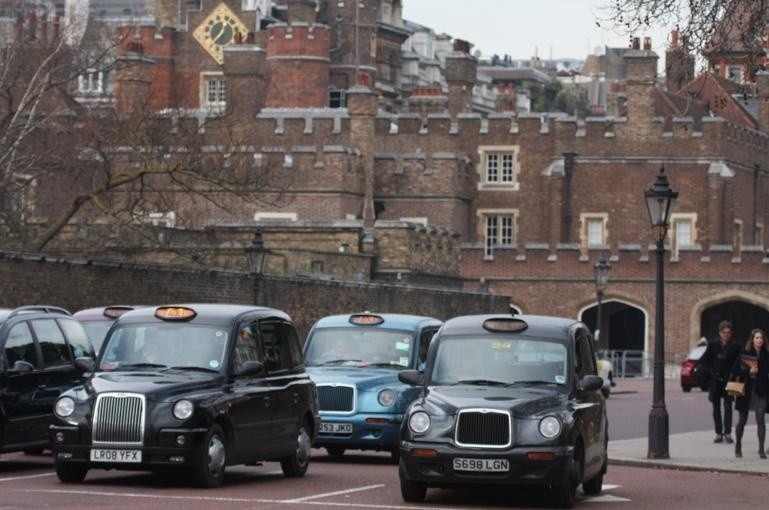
295;313;446;464
515;331;620;398
48;304;320;487
400;314;614;506
72;306;149;364
681;346;709;392
1;305;98;467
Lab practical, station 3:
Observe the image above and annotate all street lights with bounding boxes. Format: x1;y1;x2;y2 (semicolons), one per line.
641;161;680;460
591;250;614;350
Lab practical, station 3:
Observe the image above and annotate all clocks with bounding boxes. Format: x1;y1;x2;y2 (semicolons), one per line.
190;0;252;68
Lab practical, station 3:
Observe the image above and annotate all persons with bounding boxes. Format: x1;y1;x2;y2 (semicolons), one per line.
700;320;741;443
130;328;174;362
319;336;354;359
731;328;769;458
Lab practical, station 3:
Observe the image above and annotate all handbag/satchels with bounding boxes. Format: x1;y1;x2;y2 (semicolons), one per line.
725;381;745;396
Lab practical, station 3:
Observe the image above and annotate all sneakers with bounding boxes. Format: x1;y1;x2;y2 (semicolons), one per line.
715;433;732;442
